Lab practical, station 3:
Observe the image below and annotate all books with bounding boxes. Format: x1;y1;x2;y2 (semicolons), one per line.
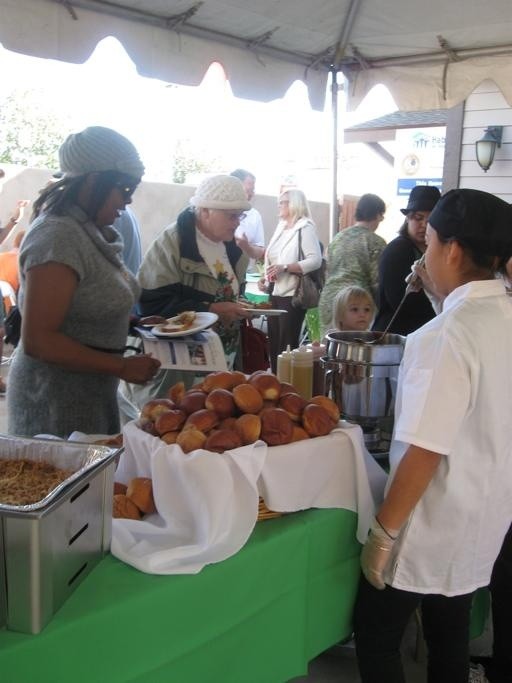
140;328;229;375
133;324;209;346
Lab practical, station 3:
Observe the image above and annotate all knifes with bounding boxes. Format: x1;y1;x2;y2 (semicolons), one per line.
142;323;184;329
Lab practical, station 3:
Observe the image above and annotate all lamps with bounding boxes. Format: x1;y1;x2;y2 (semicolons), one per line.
474;125;503;172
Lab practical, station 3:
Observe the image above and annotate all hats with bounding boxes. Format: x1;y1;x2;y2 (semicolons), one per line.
59;126;145;178
428;189;512;254
190;175;252;210
401;186;441;215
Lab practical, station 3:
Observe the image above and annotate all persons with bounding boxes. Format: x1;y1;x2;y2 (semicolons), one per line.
317;193;389;332
259;187;322;375
321;286;374;347
368;186;441;335
132;172;260;379
1;200;29;243
111;209;141;313
1;124;170;440
226;166;263;288
352;189;511;683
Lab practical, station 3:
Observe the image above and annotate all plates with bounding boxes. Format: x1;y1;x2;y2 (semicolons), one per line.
242;306;288;316
151;311;219;337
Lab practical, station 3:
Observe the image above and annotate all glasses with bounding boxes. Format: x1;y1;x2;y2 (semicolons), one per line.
116;180;134;195
210;211;247;220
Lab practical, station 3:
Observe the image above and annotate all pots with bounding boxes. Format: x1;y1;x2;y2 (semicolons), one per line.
318;330;407;457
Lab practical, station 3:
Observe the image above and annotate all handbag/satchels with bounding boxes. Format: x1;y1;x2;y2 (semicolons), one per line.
292;229;325;309
242;318;270;374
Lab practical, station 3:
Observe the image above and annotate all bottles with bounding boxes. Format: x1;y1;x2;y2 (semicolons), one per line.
277;339;326;402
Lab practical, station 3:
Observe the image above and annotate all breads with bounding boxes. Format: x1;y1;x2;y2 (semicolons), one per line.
110;475;162;522
138;367;340;459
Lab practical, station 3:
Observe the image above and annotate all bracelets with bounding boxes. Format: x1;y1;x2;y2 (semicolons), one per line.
10;217;18;224
284;264;289;272
374;515;397;541
